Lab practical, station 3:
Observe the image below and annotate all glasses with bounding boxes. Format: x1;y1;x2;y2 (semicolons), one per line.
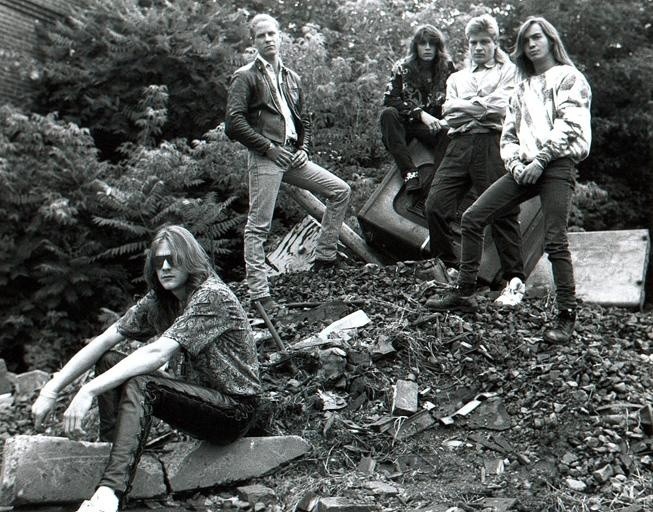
149;255;183;270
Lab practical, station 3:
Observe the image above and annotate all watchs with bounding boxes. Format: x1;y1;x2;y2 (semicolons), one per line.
266;143;276;153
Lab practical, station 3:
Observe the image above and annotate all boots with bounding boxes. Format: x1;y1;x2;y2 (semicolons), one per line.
544;308;576;345
425;281;478;312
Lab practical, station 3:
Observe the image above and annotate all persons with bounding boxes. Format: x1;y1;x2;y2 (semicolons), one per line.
29;223;264;512
424;17;593;346
424;13;528;308
382;24;460;197
225;11;352;318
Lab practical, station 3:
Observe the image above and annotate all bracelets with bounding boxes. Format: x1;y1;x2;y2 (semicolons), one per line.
40;389;58;399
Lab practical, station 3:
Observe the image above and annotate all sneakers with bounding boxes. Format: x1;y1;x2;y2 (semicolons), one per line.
404;170;422;195
314;258;349;273
76;495;119;512
251;297;283;311
494;277;526;306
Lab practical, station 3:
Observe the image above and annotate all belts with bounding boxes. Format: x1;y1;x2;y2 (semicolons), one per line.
470;128;490;134
286;139;297;147
